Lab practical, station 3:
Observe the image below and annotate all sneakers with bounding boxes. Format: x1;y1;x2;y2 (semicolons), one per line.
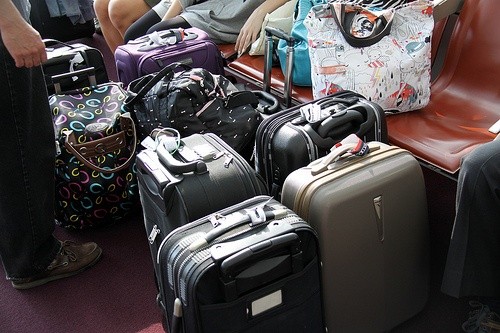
11;240;102;289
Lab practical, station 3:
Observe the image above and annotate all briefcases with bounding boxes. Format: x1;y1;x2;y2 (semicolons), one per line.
250;90;387;203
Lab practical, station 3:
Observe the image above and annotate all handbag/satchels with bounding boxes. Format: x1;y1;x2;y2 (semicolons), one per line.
277;1;325;86
304;2;434;114
48;82;142;232
126;58;265;162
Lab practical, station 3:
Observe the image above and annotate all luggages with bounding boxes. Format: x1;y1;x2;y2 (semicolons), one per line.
156;195;324;333
280;141;430;332
42;38;110;95
114;27;225;90
244;27;304;120
135;133;270;305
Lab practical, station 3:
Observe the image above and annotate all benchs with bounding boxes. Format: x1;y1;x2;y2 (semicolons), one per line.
215;0;500;183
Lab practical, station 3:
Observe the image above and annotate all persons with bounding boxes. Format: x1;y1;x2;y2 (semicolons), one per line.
439;133;500;333
0;0;103;290
122;0;288;58
93;0;161;55
